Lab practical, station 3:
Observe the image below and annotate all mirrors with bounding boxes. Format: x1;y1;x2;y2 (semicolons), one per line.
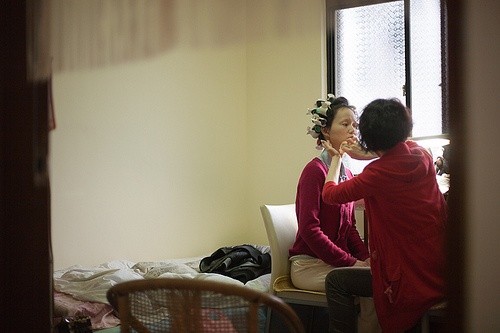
407;135;451;196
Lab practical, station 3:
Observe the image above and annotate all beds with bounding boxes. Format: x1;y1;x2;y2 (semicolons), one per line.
54;242;272;331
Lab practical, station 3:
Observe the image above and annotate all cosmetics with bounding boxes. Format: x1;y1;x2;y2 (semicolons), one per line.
339;142;348;155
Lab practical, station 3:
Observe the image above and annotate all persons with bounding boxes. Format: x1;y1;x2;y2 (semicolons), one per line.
288;97;377;333
321;98;447;333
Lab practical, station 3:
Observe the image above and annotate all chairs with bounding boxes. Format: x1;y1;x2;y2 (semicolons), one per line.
105;277;307;333
260;203;360;333
